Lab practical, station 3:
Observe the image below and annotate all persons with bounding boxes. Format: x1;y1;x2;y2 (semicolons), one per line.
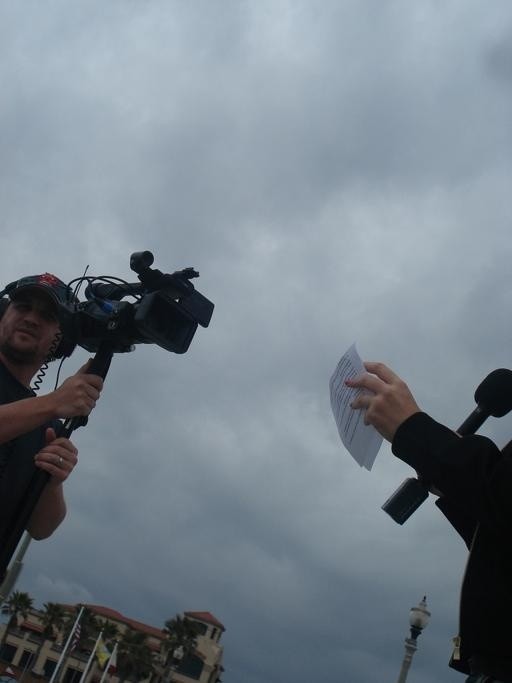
343;358;511;682
1;272;106;587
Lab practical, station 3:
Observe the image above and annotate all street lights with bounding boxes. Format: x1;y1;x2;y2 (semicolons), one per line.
19;646;37;682
396;594;432;682
162;644;186;682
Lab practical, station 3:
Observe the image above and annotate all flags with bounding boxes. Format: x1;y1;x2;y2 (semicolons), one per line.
108;641;119;675
70;605;84;652
92;635;113;670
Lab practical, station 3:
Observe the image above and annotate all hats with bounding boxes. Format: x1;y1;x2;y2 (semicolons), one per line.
10;273;72;306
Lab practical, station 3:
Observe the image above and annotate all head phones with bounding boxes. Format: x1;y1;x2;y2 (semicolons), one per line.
0;274;77;359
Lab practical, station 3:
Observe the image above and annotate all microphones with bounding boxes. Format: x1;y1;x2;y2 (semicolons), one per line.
381;368;512;525
